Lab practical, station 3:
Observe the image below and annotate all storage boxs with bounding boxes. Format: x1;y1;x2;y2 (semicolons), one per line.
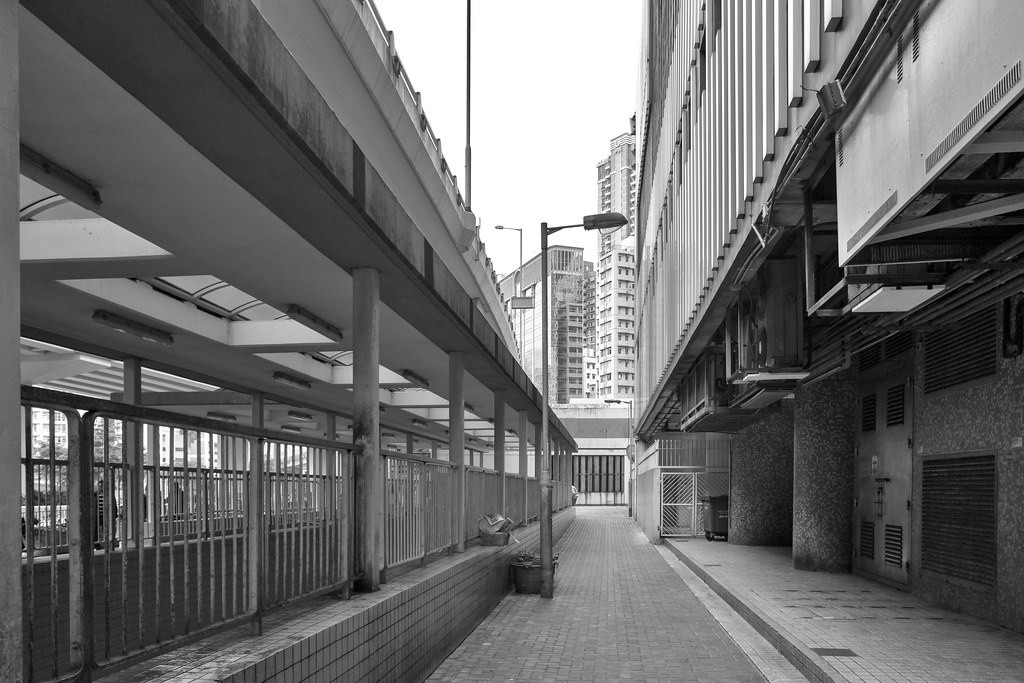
499;518;515;533
479;531;508;546
510;561;559;593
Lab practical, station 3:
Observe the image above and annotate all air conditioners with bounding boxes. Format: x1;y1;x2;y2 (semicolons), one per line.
844;262;928;313
754;258;798;368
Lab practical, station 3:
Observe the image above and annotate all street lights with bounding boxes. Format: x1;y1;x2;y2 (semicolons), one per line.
460;3;473;213
495;225;524;371
534;212;629;599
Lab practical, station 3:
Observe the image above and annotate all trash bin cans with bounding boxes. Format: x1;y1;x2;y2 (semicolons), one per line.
700;495;728;542
511;563;556;594
